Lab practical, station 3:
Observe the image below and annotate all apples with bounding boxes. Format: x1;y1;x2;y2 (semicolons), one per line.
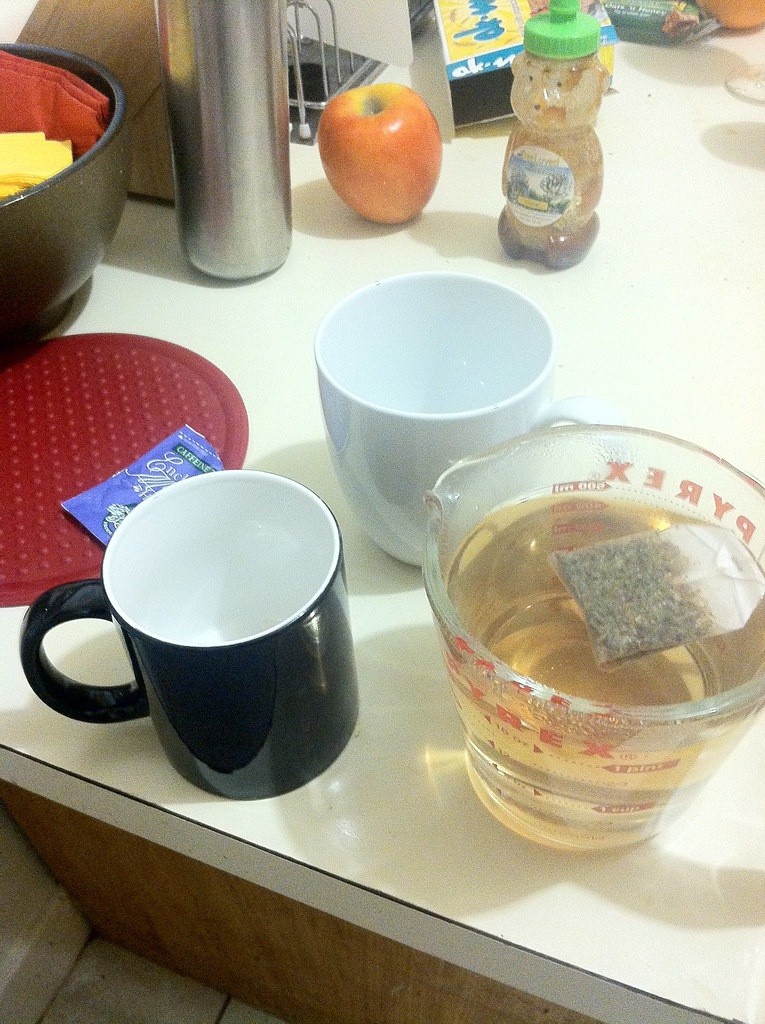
696;0;765;29
317;85;442;225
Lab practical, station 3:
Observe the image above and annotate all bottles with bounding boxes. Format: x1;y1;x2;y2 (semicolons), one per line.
494;0;613;270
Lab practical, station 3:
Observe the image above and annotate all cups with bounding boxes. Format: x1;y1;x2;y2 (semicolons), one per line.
16;469;361;804
312;271;557;568
414;422;765;850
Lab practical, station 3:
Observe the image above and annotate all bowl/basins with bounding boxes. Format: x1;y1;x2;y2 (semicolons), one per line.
0;42;131;344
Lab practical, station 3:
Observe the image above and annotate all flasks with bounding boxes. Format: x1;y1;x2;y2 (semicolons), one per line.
152;0;294;279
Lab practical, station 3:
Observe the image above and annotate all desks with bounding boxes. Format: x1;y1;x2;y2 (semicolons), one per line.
0;0;765;1024
